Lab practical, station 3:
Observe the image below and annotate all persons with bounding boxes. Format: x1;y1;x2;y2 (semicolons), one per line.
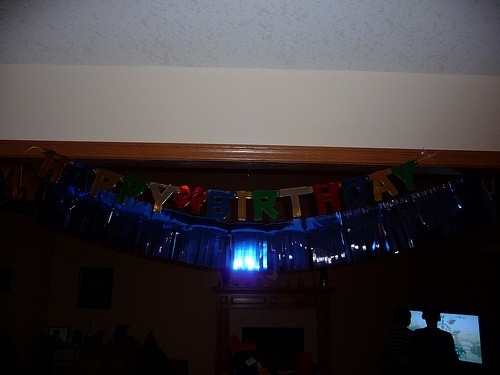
410;302;459;375
255;351;277;375
382;304;416;375
49;328;62;343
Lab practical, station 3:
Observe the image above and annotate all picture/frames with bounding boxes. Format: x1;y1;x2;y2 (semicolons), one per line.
218;268;261;288
48;326;70;344
264;268;326;288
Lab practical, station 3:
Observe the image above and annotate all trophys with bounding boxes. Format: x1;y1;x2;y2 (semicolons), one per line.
83;319;96;346
